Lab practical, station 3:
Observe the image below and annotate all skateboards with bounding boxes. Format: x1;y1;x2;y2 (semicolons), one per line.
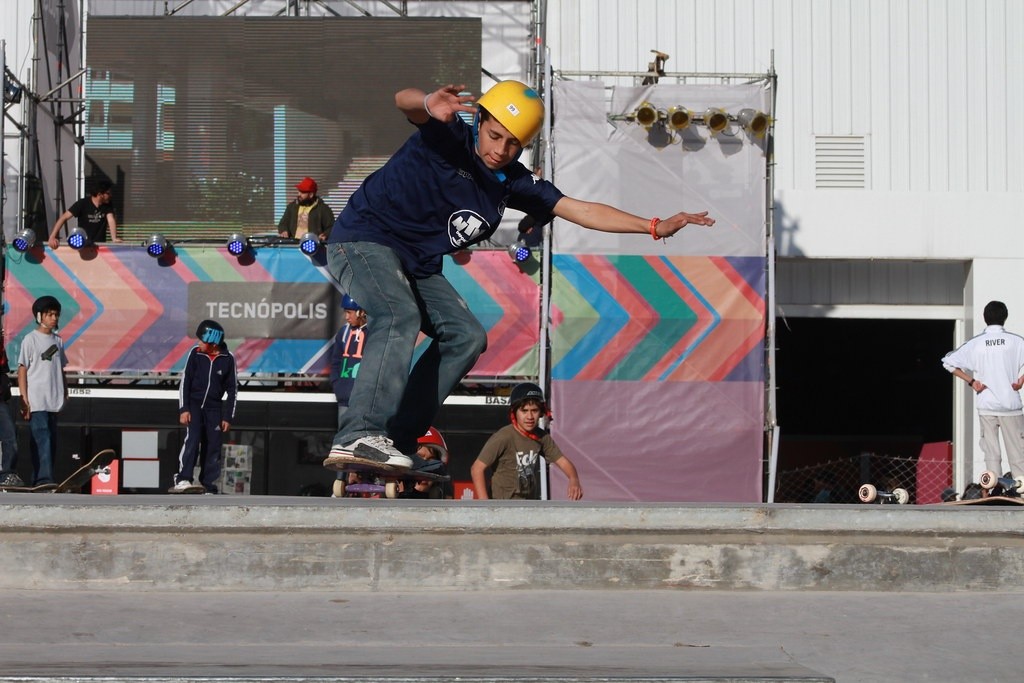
0;483;59;493
322;454;452;498
53;448;115;494
168;486;207;495
859;470;1024;506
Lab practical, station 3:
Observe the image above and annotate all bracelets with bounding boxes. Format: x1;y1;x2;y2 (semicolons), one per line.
969;379;975;386
650;218;660;240
424;93;432;118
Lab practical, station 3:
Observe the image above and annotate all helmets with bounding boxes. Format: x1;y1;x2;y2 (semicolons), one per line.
195;320;228;353
475;79;544;147
941;489;959;502
510;382;543;406
340;294;361;311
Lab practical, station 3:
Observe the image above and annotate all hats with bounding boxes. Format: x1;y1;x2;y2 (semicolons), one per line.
293;177;318;193
417;425;449;464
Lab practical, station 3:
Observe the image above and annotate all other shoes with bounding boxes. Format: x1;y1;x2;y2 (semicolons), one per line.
174;480;191;490
0;473;25;486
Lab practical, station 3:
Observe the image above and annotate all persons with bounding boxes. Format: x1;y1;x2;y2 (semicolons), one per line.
329;294;368;483
0;297;71;494
173;319;238;495
471;383;584;500
349;426;449;499
940;301;1024;497
811;472;847;504
327;80;715;466
49;179;123;250
278;177;335;239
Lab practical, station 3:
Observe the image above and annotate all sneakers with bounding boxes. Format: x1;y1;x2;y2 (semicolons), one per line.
329;434;415;467
